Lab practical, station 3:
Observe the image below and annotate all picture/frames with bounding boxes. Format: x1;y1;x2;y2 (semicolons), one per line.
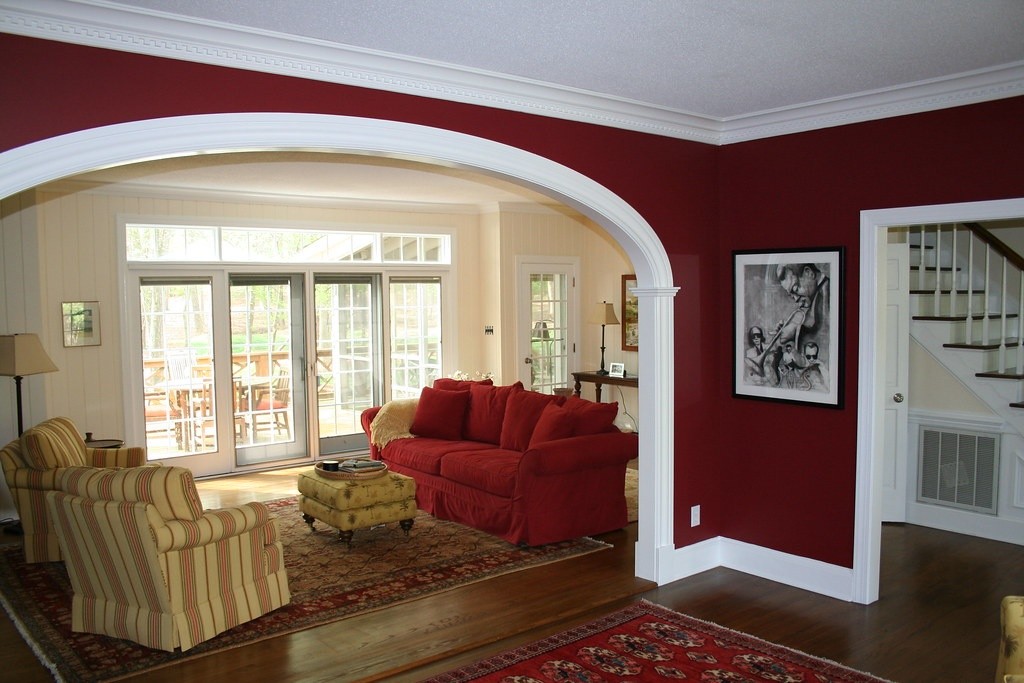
609;362;625;377
729;245;847;410
621;274;638;352
60;301;101;348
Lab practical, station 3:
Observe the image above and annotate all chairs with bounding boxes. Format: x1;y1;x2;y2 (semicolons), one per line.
144;368;291;453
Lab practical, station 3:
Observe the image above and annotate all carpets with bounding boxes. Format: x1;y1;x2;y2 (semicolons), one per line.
418;598;897;683
0;493;613;683
625;468;639;523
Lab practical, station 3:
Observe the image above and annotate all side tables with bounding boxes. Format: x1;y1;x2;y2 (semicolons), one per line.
85;439;126;449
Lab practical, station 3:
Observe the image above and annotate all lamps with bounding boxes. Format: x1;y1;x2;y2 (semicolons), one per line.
0;332;60;438
586;300;620;375
533;322;554;394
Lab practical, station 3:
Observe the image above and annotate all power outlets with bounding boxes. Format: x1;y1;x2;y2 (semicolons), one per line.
691;505;700;528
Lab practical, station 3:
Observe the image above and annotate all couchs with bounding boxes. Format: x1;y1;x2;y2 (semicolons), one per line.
360;398;638;547
44;464;291;653
0;414;161;564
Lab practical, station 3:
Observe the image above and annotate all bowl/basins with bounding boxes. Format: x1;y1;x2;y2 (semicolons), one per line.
554;388;573;395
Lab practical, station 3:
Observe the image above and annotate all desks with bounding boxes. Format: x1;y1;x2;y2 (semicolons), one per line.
155;376;277;450
570;370;638;403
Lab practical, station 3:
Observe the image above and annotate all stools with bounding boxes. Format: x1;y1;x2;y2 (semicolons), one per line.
297;469;418;550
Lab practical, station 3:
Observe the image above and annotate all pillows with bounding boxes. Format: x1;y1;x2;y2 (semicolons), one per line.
528;398;576;446
562;394;619;437
433;378;493;415
409;386;470;441
499;385;567;452
463;380;525;445
